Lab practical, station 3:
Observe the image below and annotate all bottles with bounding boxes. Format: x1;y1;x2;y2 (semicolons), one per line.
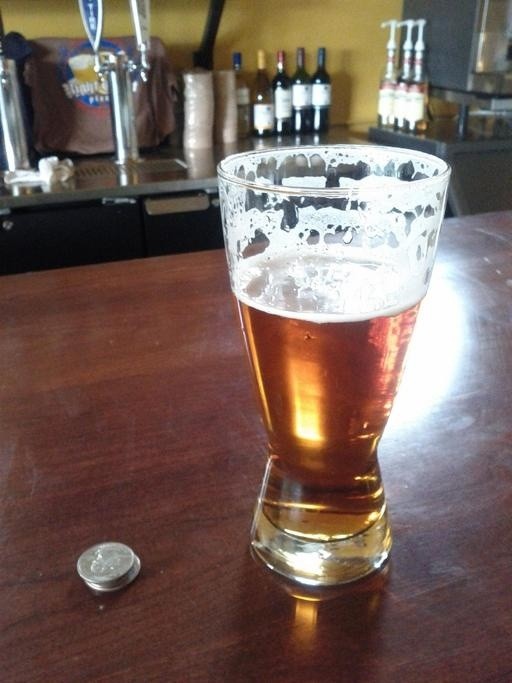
249;48;275;138
271;49;291;135
396;17;415;131
291;47;312;135
312;46;332;135
408;18;431;137
232;51;252;140
376;17;397;128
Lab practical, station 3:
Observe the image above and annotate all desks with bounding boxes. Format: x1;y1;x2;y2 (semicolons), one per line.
4;207;510;678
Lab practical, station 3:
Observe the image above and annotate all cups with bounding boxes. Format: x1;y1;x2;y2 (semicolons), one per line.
217;142;456;591
